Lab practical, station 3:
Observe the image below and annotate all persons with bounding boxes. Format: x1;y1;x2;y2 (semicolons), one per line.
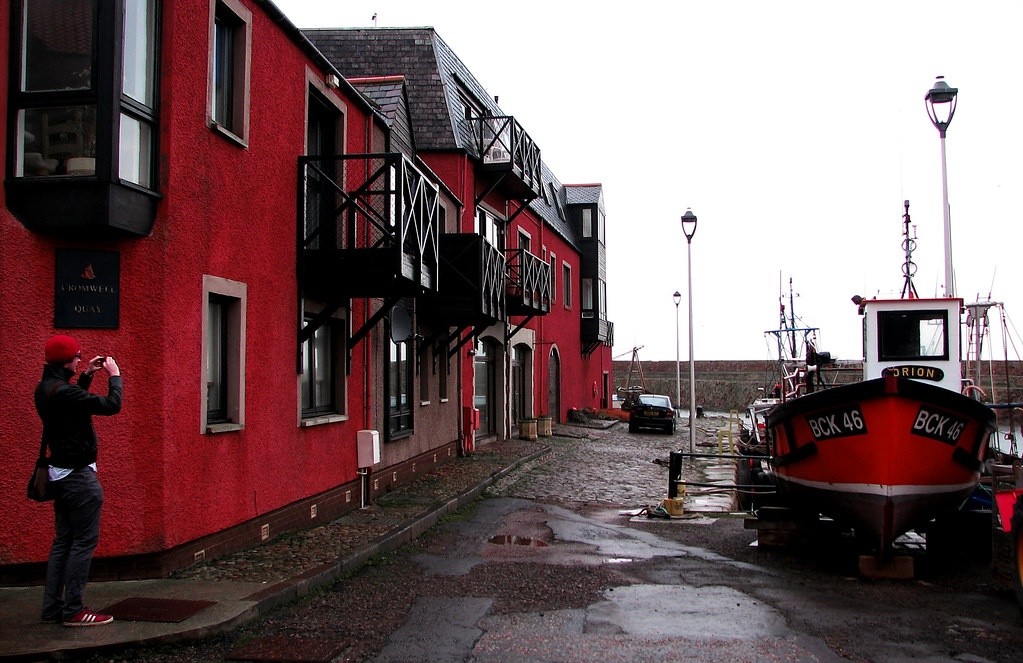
34;334;122;626
772;383;781;398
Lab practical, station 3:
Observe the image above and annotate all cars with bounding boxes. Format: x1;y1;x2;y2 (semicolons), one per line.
629;395;676;434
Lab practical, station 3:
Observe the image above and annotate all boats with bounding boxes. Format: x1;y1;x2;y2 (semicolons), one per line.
742;202;1023;577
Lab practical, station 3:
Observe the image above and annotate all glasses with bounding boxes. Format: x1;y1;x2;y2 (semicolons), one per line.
74;354;81;358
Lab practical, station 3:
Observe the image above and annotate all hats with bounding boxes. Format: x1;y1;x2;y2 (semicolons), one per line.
45;335;79;363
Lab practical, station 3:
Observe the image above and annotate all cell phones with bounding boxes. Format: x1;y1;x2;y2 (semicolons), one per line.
97;359;106;368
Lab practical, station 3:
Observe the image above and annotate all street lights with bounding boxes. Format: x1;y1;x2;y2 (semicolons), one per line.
681;206;698;460
925;72;960;297
672;290;682;417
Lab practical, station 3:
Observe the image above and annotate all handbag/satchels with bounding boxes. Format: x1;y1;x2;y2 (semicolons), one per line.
27;456;54;502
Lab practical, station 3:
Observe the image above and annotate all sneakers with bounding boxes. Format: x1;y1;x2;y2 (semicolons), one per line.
41;609;63;623
63;605;114;626
